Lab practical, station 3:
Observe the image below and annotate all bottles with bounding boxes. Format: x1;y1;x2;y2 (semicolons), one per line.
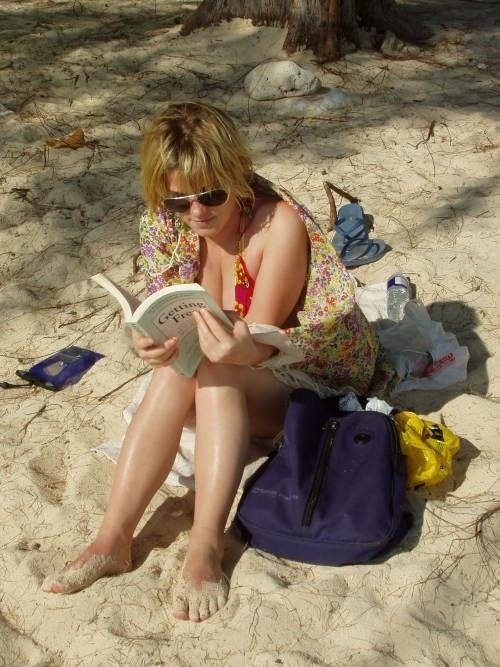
385;270;412;321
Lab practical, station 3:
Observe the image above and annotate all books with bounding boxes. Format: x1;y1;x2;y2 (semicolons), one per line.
89;271;233;379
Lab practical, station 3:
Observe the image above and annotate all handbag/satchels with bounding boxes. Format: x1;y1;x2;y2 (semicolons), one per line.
235;387;406;566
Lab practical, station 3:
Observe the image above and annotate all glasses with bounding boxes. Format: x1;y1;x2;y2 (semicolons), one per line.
159;185;230;211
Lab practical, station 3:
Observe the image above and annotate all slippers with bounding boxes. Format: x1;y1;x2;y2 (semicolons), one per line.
339;237;385;266
331;204;367;256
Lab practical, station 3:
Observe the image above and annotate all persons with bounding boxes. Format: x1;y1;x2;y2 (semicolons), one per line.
41;102;395;624
